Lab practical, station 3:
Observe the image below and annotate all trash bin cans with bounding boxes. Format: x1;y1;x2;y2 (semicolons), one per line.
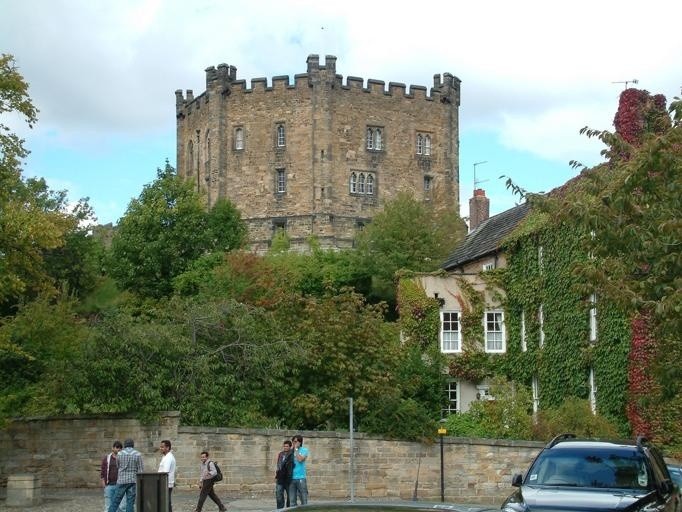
133;471;171;512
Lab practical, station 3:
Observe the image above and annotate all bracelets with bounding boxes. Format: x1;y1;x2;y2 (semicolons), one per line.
295;448;298;450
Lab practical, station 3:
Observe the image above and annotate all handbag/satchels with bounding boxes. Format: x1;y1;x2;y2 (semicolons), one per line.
207;461;222;482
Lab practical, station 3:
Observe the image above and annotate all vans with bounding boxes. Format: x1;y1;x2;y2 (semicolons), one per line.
500;434;682;511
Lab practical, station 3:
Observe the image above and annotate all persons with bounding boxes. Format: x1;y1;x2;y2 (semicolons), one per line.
195;452;227;512
108;439;142;512
275;440;293;509
157;439;177;512
100;441;127;512
289;434;309;507
